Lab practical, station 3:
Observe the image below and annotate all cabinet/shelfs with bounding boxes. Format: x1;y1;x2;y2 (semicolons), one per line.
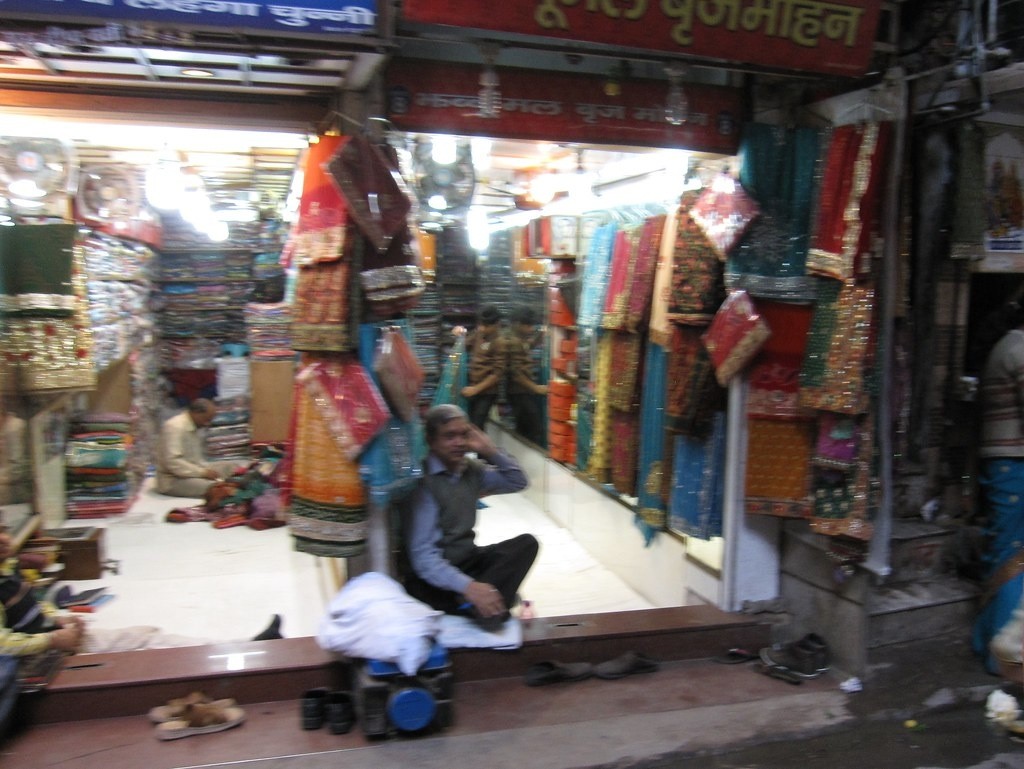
0;221;282;395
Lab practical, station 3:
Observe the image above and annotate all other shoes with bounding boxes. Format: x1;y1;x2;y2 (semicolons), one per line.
525;657;595;686
758;630;833;679
298;684;326;730
593;648;659;680
328;691;357;735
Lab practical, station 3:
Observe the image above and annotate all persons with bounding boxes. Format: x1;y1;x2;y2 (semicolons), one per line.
402;404;539;631
452;305;505;432
0;580;281;657
974;287;1024;674
157;398;221;497
505;306;548;449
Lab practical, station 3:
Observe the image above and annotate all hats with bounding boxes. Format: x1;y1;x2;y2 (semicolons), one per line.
477;305;510;325
510;304;539;325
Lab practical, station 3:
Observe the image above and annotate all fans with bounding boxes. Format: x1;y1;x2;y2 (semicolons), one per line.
412;143;476;231
77;165;140;222
0;137;68;200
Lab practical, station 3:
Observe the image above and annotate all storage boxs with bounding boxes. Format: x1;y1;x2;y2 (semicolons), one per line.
21;528;106;580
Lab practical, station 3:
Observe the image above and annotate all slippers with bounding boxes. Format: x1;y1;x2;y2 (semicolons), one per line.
713;644;762;666
753;662;802;686
145;690;248;743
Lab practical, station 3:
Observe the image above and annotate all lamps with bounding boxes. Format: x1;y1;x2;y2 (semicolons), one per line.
477;42;503;118
661;62;693;127
